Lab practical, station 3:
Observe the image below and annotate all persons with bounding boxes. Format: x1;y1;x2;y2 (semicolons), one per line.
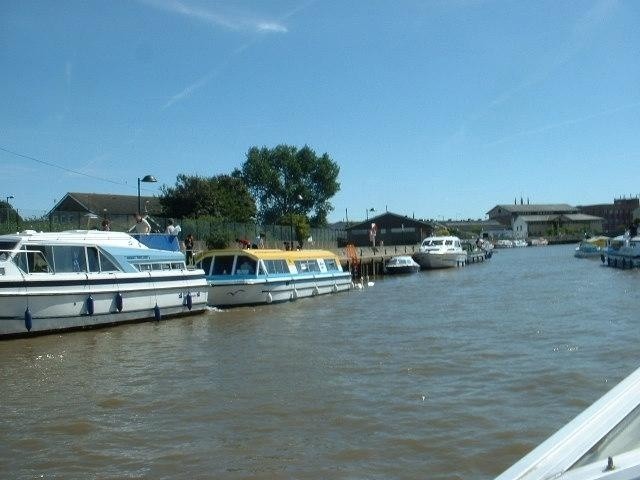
475;238;484;251
251;234;266;249
133;213;151;234
283;241;292;250
165;218;175;234
102;220;110;231
184;234;195;265
297;241;304;250
236;238;250;250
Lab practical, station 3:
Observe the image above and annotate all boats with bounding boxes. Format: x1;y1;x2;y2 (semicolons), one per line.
413;236;468;268
575;234;610;258
193;249;352;310
600;230;640;268
385;255;421;275
0;228;209;335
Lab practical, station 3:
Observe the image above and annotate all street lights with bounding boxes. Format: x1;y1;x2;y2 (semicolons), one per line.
366;208;376;246
137;175;157;215
6;195;14;233
289;195;304;250
